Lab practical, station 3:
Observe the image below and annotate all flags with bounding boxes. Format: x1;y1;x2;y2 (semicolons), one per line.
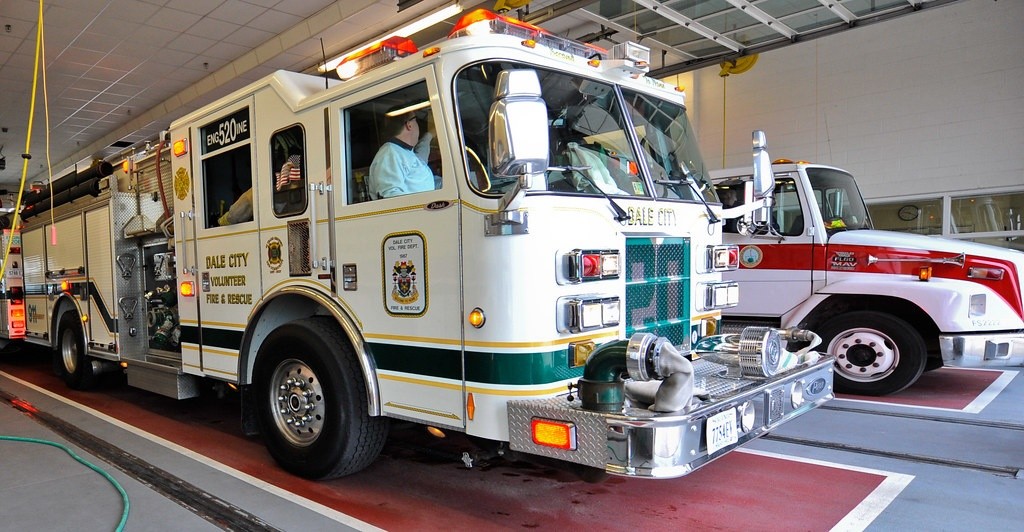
275;154;301;192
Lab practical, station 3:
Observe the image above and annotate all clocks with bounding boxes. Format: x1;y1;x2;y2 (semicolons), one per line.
897;204;919;221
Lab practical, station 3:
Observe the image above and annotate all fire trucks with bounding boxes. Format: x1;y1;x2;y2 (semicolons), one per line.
679;157;1023;398
0;7;837;484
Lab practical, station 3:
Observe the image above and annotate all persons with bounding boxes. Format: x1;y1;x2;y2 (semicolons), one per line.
716;189;740;233
367;103;436;200
557;136;589;156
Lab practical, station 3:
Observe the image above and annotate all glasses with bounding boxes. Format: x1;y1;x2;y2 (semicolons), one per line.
402;114;419;125
